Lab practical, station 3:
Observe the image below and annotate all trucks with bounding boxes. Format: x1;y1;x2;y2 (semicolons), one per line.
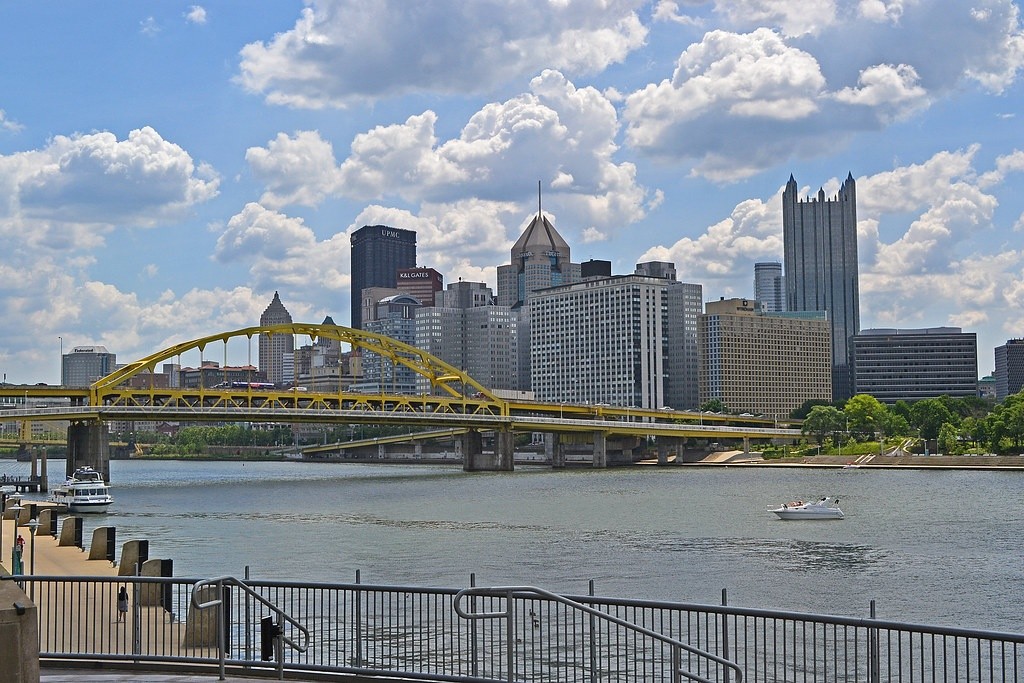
288;387;308;392
395;391;416;396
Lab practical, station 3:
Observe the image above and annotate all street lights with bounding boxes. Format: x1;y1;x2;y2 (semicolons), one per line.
57;336;62;387
8;504;26;549
0;489;7;562
23;519;42;604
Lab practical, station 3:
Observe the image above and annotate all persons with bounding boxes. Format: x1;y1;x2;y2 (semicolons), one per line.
116;586;129;623
17;535;26;556
796;502;803;506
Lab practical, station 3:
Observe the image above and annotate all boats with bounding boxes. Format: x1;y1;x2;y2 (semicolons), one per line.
767;499;846;520
48;466;113;514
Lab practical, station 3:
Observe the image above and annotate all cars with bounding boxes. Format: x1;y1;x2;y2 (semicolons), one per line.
685;409;767;418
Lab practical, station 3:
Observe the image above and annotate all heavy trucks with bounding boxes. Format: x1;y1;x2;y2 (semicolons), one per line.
214;381;275;389
473;389;534;401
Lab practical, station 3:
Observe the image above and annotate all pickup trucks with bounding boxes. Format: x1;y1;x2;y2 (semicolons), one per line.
595;402;610;407
660;406;674;411
